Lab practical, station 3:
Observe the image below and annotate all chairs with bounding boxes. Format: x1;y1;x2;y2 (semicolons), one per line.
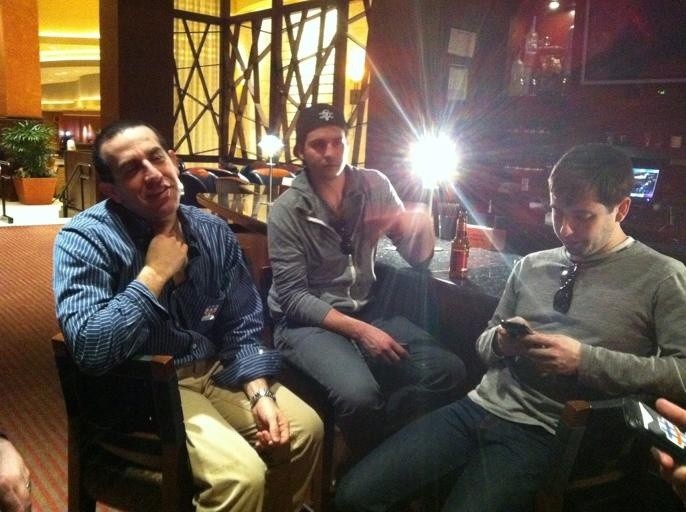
259;263;338;512
526;393;656;511
51;332;199;511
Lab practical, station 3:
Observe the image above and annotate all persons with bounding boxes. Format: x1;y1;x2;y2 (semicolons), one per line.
334;143;686;512
264;103;468;493
649;398;686;503
0;431;33;512
52;122;325;511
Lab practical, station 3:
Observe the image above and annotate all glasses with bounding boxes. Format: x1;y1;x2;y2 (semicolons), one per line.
553;262;579;312
335;219;353;255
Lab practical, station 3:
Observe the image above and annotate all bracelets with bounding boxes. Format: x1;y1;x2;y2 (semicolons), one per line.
249;389;276;405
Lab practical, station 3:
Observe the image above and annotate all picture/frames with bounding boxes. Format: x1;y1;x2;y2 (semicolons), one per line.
571;0;684;90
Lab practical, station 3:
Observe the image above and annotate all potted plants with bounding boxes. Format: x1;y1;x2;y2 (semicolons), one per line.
0;117;59;205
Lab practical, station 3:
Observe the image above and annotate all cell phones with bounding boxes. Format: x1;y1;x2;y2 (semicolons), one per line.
622;395;686;457
500;317;533;335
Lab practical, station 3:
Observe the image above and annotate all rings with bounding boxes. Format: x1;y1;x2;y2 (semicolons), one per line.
26;482;30;488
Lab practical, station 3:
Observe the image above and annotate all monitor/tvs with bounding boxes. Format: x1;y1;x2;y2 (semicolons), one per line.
626;167;663;208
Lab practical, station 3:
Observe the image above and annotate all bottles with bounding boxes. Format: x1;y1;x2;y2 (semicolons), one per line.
449;210;469;279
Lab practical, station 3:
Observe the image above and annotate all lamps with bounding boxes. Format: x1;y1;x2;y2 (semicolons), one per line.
257;134;285;206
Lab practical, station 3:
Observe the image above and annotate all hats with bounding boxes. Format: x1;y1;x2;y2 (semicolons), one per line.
293;103;345;157
